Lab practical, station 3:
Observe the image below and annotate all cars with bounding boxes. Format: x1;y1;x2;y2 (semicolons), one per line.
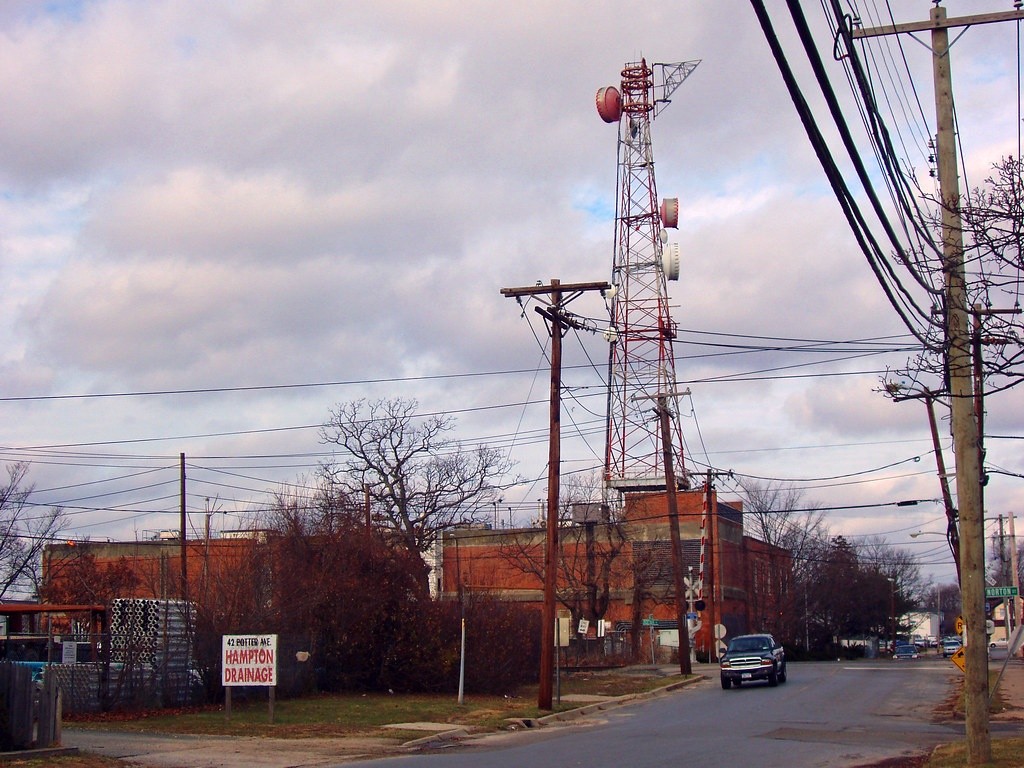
53;641;204;704
988;638;1009;649
878;643;892;654
890;645;922;661
929;640;940;648
940;637;962;645
915;638;928;648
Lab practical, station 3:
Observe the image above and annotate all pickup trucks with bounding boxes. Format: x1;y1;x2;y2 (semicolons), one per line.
716;634;788;689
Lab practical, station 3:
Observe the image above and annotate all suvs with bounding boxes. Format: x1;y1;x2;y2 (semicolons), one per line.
943;640;962;658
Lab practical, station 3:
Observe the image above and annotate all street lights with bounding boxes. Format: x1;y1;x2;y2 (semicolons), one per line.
449;532;461;618
493;502;496;529
888;577;896;647
507;507;513;528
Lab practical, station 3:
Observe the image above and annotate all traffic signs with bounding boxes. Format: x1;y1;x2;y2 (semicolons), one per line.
641;619;659;626
986;587;1018;597
